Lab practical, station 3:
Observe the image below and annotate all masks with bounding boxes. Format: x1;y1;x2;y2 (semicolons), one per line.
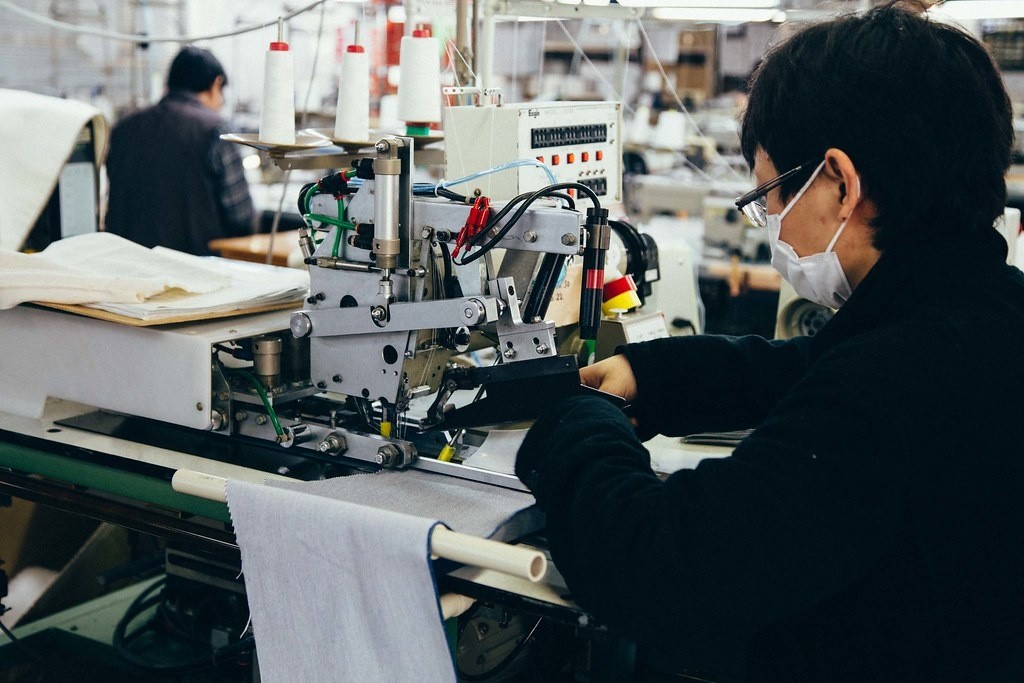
766;160;861;309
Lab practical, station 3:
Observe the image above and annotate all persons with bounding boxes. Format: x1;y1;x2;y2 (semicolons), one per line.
515;0;1024;683
103;46;261;258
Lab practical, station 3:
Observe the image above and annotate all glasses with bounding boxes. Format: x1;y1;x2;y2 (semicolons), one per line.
735;165;806;227
